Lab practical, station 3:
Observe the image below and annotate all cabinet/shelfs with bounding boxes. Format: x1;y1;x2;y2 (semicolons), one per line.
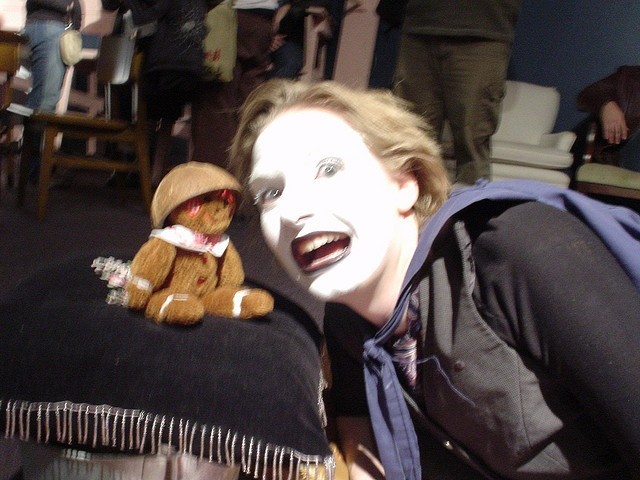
1;28;31;205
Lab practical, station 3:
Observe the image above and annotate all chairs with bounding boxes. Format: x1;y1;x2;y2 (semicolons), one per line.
579;97;636;214
34;56;152;222
434;76;576;186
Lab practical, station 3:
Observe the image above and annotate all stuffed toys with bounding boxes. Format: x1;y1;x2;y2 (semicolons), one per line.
122;160;275;329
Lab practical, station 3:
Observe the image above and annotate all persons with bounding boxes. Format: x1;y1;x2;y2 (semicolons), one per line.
231;1;306;108
136;0;208;215
99;1;135;142
16;0;82;219
574;65;640;168
392;0;517;183
227;78;639;479
195;2;278;228
